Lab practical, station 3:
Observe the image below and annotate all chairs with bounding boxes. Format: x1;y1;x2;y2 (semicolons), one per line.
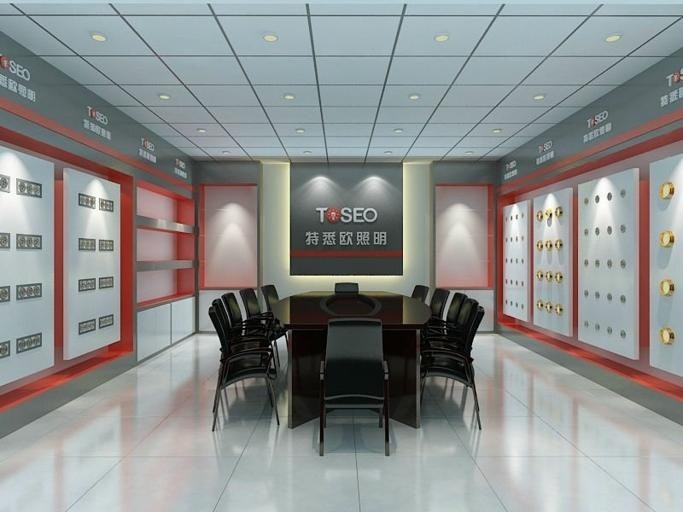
206;282;485;457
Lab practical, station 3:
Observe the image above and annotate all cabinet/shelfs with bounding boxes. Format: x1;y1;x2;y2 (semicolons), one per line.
138;168;197;364
198;183;257;289
435;184;493;290
